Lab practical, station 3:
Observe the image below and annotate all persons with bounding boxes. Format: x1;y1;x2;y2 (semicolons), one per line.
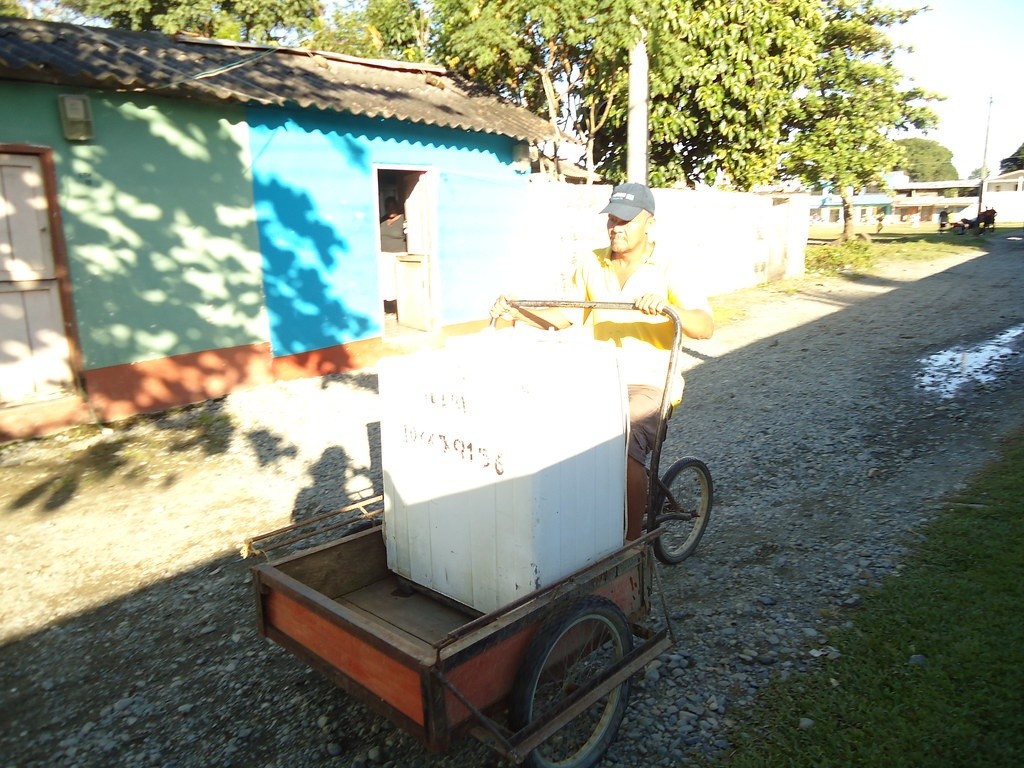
491;182;715;614
379;197;406;321
874;208;949;234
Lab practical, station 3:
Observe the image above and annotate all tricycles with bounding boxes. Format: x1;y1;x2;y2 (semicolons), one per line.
245;301;715;768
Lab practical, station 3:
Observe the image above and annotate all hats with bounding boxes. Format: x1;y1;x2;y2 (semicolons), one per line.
598;183;655;221
